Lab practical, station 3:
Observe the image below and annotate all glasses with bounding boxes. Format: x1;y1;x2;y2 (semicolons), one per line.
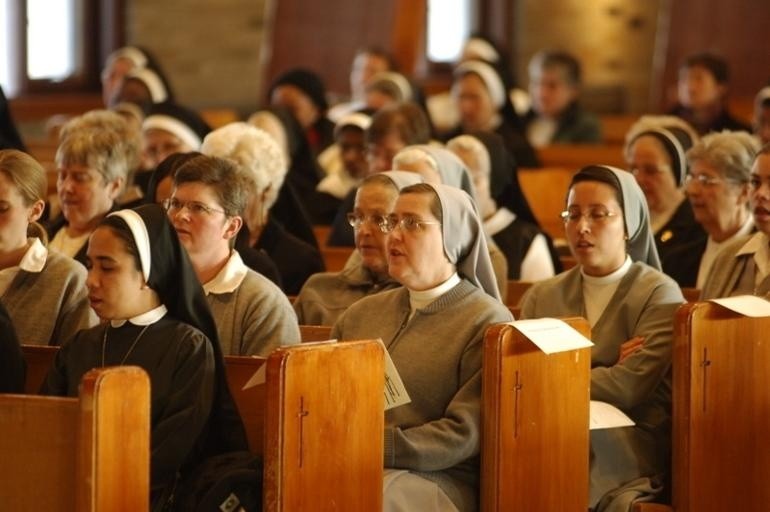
684;171;743;187
558;205;617;227
158;196;228;217
345;211;440;234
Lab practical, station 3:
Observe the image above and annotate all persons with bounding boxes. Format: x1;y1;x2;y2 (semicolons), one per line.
666;50;752;137
753;85;770;156
517;166;688;512
0;25;602;512
624;115;770;301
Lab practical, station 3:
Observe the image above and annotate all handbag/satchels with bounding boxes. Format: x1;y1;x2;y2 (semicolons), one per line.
173;449;263;512
